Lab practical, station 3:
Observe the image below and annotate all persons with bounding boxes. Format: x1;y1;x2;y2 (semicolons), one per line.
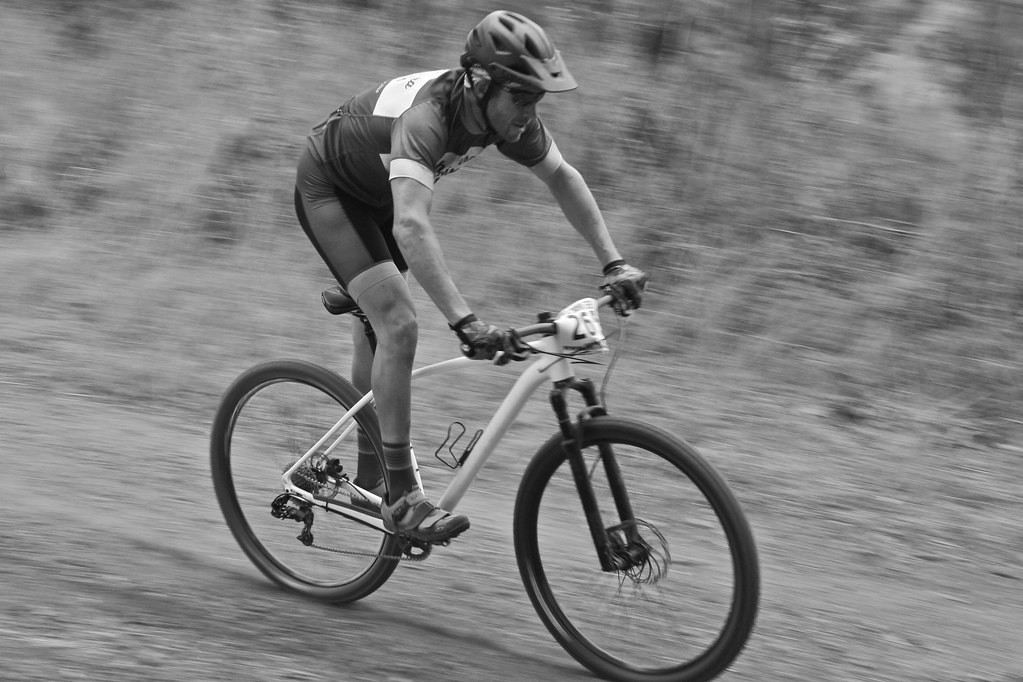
293;11;648;540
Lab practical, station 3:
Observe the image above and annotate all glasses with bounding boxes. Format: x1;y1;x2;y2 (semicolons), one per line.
489;76;546;106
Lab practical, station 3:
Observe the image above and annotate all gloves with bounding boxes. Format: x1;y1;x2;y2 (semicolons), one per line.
456;316;529;367
601;258;648;317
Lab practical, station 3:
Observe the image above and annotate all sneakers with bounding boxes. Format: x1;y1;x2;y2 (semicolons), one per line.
350;476;390;513
381;490;470;543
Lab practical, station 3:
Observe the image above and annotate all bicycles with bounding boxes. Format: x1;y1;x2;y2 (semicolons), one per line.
208;290;761;682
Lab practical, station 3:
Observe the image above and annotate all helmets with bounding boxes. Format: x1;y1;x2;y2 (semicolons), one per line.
459;10;579;95
